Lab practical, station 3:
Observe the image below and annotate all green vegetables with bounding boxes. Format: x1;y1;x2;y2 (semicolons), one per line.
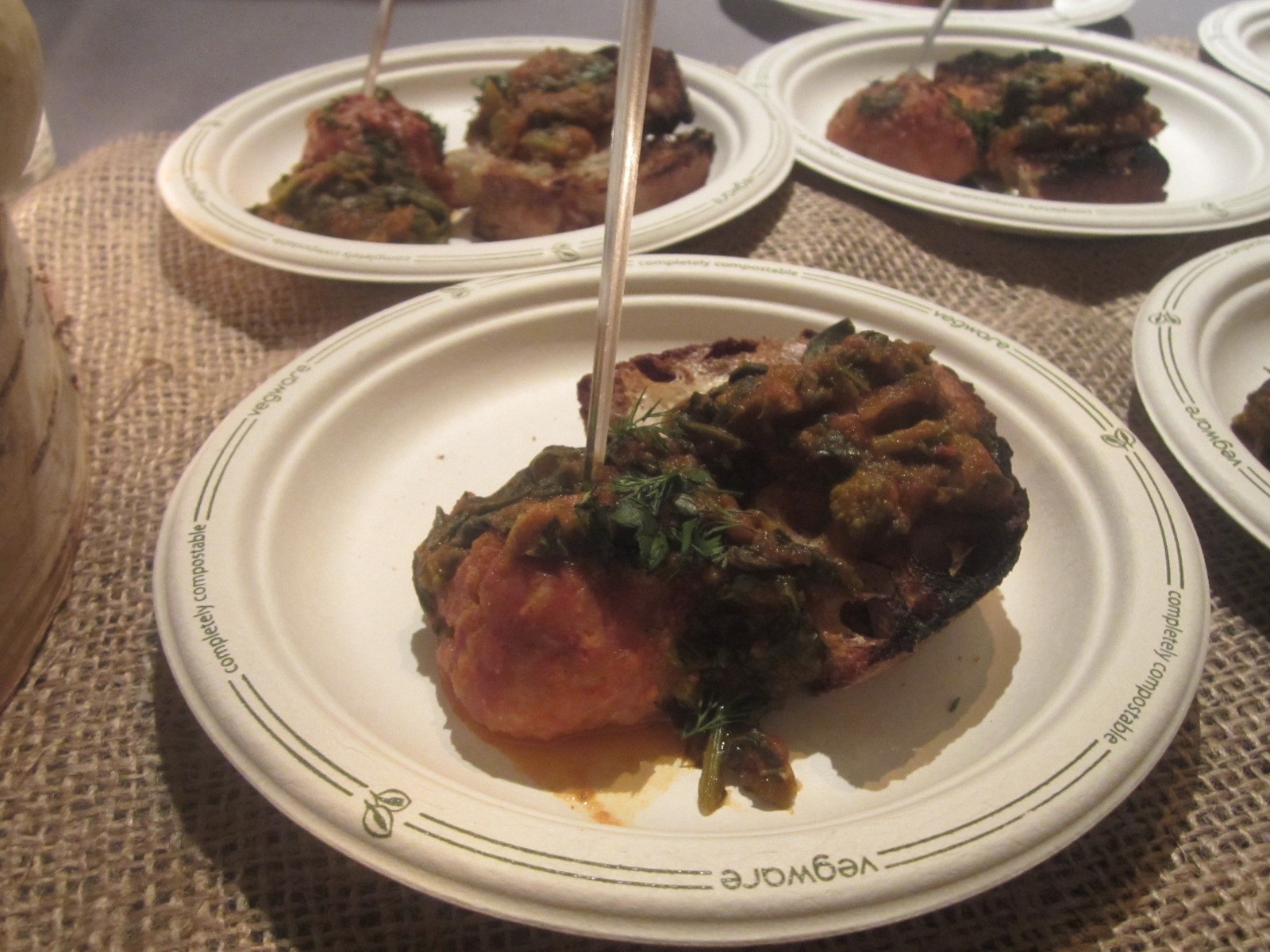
517;314;862;813
249;51;614;240
862;46;1149;172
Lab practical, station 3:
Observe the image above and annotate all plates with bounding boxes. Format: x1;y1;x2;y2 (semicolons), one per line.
153;34;795;280
766;1;1144;42
1195;1;1270;96
145;255;1212;945
1131;235;1268;574
728;21;1270;234
